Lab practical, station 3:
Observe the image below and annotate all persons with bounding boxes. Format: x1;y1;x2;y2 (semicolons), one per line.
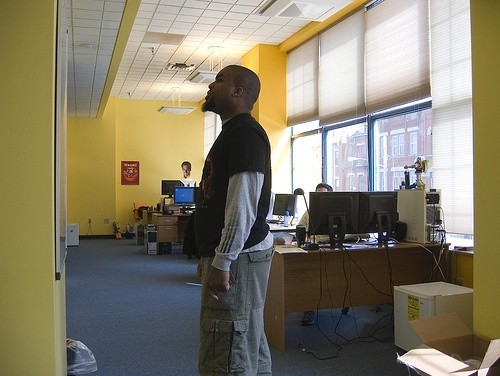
181;161;196;188
299;182;333;326
294;188;304;195
194;65;275;376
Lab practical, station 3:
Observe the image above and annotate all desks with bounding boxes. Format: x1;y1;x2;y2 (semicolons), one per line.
263;243;451;351
139;208;195;254
267;222;298;234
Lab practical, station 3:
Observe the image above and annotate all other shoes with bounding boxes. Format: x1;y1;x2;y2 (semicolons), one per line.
301;311;314;324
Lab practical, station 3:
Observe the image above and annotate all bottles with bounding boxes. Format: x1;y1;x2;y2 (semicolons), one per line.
285;210;289;227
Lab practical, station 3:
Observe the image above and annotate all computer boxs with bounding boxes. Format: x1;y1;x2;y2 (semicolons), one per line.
393;189;443;244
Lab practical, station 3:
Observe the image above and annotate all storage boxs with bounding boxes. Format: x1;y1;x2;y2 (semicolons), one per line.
395;283;474;352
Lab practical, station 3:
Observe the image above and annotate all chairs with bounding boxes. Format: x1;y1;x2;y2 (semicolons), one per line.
132;201;147;219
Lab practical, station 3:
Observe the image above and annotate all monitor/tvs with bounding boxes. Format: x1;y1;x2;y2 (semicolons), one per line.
273;191;399;249
162;180;198;205
